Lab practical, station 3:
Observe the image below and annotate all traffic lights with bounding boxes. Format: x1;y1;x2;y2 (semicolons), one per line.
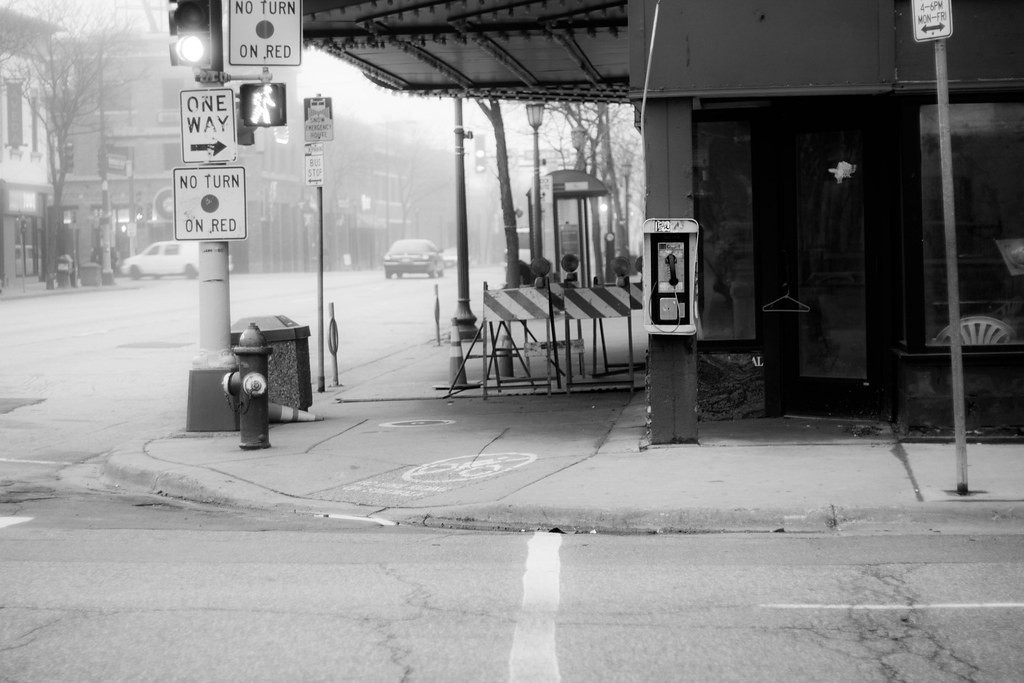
240;82;286;128
169;2;213;66
474;133;485;173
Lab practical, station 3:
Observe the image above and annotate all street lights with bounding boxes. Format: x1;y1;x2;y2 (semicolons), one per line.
525;100;545;282
622;160;631;264
570;125;592;289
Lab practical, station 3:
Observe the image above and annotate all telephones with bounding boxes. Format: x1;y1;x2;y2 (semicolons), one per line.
641;218;699;336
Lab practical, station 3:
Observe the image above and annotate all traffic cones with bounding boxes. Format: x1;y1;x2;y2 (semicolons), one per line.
268;401;324;424
494;328;526;382
435;318;482;389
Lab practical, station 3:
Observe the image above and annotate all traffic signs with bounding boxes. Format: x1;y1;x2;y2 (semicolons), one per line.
180;88;237;164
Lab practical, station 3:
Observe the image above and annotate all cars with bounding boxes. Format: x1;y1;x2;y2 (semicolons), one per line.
384;238;446;277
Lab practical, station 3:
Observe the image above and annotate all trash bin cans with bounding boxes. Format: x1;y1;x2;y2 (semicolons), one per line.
79;262;102;286
230;315;313;412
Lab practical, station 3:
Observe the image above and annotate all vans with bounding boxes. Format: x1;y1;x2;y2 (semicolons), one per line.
119;240;234;279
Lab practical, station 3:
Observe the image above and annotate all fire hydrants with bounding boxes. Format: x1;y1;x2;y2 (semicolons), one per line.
224;323;272;450
